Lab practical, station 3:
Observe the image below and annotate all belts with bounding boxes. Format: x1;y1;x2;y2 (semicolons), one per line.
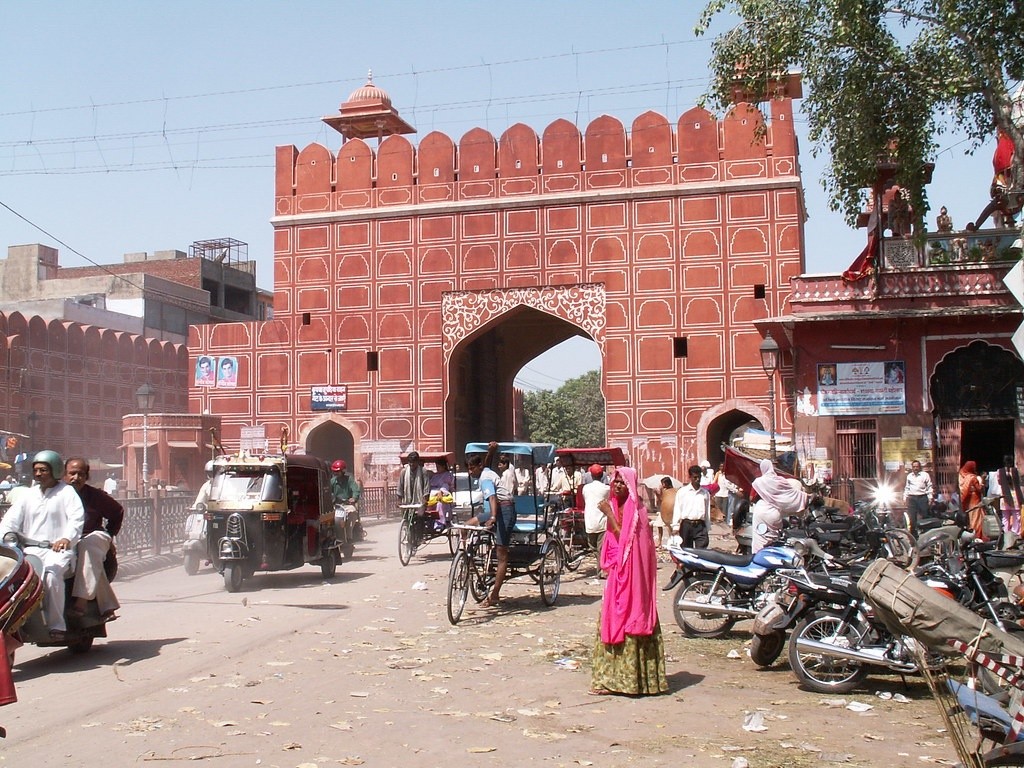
496;500;515;506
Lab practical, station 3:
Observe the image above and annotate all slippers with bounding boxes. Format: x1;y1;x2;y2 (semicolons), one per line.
479;597;499;608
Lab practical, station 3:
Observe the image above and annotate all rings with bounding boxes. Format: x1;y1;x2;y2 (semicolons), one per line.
60;544;63;546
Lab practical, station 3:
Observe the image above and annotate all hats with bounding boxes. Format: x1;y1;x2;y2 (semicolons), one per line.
590;464;603;477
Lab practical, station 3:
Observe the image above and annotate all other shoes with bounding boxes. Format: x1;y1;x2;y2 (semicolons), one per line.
71;607;88;617
104;612;119;623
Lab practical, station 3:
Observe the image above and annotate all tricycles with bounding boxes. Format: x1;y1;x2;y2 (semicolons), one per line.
446;442;562;625
397;452;460;566
555;447;629;571
857;559;1024;768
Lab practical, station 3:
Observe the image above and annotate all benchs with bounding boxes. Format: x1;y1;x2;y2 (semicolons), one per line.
483;494;545;533
565;484;586;517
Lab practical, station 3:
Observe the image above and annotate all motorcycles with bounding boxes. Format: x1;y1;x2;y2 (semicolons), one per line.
206;452;342;592
662;500;1024;693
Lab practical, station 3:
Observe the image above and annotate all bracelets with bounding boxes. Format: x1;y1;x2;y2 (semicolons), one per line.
490;516;496;519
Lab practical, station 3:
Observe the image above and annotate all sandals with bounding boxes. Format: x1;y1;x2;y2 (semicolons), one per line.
49;630;65;641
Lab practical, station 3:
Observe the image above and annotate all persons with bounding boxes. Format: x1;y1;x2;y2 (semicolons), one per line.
218;358;237;387
587;466;671;696
396;451;454;544
328;459;361;545
64;457;124;623
653;465;711;591
581;463;611;580
0;449;85;643
455;463;463;472
103;472;119;499
196;357;215;385
497;454;609;512
0;474;32;503
902;454;1024;542
700;462;744;528
460;452;517;608
749;459;814;553
184;459;229;512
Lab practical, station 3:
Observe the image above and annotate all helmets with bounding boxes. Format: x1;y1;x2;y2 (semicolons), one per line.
204;460;215;472
331;460;346;471
32;450;65;479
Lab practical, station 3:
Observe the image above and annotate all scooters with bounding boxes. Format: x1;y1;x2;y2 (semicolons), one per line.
0;530;120;657
332;493;360;561
183;502;212;575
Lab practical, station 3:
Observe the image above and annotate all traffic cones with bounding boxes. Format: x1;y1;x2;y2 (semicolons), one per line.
0;631;18;706
